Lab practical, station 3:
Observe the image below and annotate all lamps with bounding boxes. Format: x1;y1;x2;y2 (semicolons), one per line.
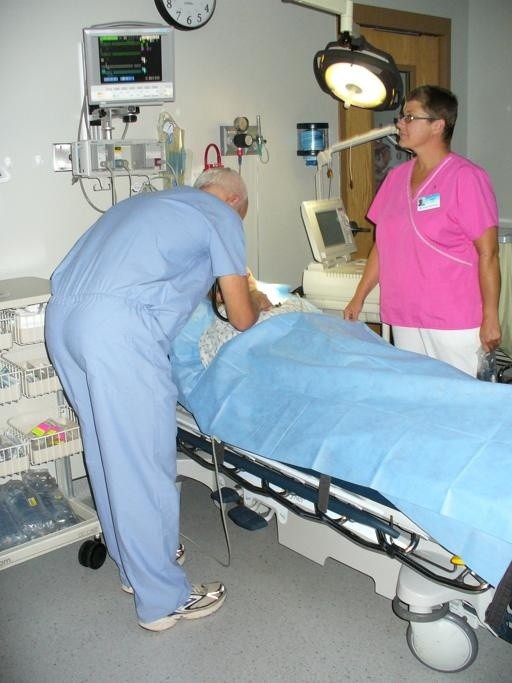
313;39;403;111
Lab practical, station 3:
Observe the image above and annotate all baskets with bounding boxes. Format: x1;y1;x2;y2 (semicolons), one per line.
0;304;83;476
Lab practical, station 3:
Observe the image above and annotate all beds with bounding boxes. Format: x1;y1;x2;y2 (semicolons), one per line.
174;396;497;673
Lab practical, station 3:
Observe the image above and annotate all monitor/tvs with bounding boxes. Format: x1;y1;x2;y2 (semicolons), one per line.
82;20;175;108
301;198;358;263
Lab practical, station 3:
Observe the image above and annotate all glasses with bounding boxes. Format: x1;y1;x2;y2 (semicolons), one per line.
398;113;435;123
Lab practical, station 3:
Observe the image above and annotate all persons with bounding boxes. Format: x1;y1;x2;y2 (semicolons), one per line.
43;168;269;629
199;268;321;372
344;85;503;377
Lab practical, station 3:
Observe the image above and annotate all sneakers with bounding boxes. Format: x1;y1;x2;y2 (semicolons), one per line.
122;544;185;594
139;582;227;632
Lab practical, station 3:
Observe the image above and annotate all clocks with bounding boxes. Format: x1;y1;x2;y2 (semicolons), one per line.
154;0;219;31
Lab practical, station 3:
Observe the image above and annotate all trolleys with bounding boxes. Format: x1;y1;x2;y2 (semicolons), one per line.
1;276;107;570
